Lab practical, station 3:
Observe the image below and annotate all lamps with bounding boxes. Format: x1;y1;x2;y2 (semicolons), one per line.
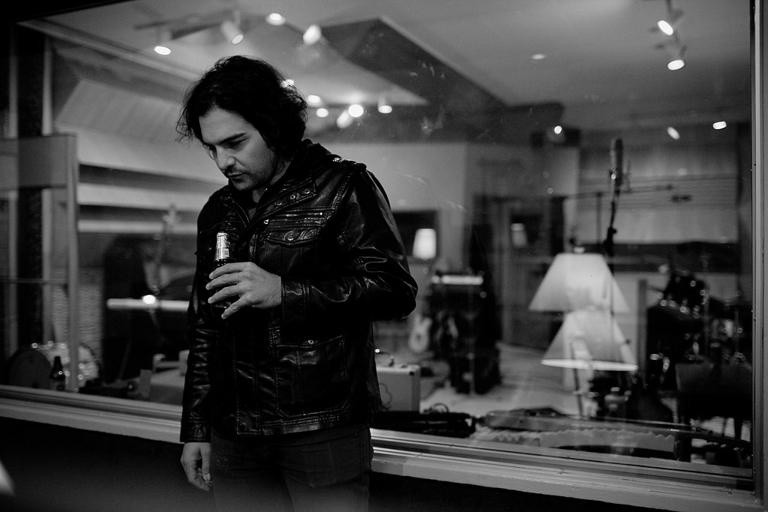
530;246;640;423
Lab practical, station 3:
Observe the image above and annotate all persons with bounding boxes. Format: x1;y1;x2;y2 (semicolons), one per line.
178;54;419;510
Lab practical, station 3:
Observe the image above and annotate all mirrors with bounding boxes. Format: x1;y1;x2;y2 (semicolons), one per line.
1;0;764;507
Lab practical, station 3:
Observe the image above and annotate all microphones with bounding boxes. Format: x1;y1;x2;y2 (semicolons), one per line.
609;137;625;187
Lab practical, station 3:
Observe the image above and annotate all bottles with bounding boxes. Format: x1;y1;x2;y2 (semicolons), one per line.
208;229;237;311
